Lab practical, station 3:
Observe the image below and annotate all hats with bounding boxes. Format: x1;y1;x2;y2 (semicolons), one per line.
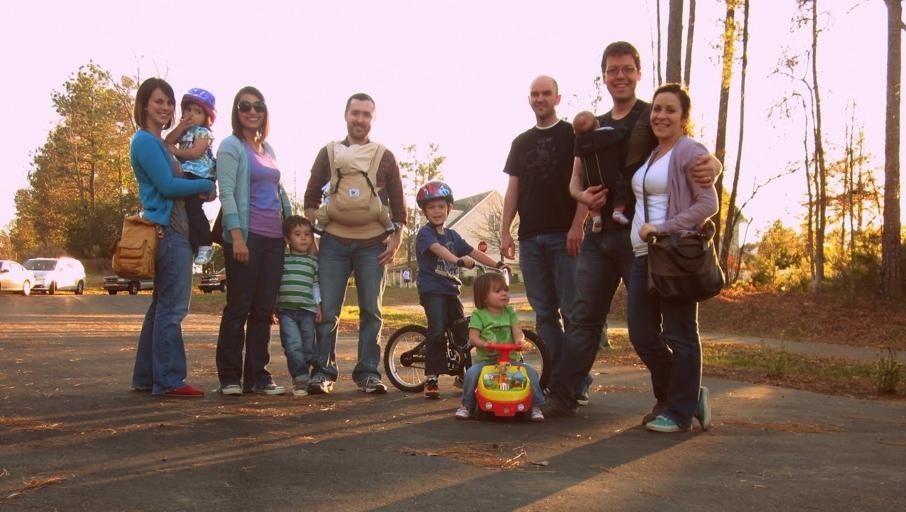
183;87;217;122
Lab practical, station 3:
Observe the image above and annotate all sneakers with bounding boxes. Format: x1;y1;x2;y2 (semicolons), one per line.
163;383;204;396
453;376;464;388
642;401;668;424
540;397;576;416
424;380;440;398
645;406;692;432
310;375;333;393
222;385;242;396
292;384;308;397
578;390;589;407
455;405;469;419
260;381;285;395
531;406;544;420
693;385;711;430
356;377;387;394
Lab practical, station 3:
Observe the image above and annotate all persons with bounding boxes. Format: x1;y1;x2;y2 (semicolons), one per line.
127;77;205;398
541;38;724;425
455;273;545;424
415;181;512;396
571;110;630;232
401;264;412;287
312;143;400;239
273;214;324;401
216;85;292;396
627;82;712;435
304;93;408;395
165;86;216;266
499;75;594;406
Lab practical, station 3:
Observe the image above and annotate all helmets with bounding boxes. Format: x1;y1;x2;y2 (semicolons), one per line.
416;180;454;207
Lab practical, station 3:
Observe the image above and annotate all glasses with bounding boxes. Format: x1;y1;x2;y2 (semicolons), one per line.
236;100;265;113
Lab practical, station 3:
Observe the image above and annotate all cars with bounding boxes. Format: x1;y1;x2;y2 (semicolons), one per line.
0;260;35;295
103;274;154;295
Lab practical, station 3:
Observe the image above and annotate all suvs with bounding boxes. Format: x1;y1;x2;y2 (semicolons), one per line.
199;265;227;293
22;256;85;294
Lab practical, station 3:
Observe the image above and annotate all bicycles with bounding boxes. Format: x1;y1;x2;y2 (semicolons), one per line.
383;260;550;401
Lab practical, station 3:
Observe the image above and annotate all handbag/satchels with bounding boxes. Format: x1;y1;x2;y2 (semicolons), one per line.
643;148;727;303
112;213;153;278
